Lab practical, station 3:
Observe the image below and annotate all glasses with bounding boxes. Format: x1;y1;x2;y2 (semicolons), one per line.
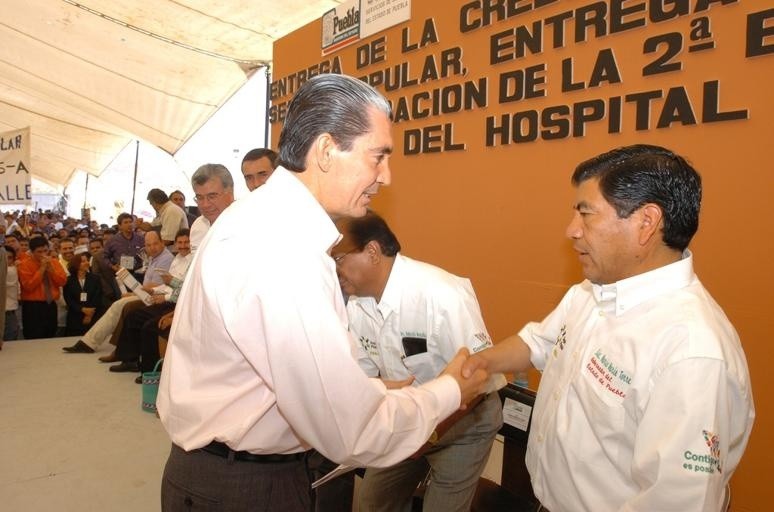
193;192;228;204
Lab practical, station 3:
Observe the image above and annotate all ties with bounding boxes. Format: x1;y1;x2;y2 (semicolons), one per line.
42;271;53;304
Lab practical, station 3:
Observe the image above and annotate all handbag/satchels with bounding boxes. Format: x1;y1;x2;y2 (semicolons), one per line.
141;359;163;413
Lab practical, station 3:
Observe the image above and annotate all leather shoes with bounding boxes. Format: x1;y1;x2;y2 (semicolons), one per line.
63;340;94;353
99;354;117;362
110;361;140;372
135;376;142;384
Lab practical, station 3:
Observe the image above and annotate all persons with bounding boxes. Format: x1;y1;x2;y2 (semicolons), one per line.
186;162;236;256
154;73;487;511
461;143;755;511
239;145;279;193
329;207;508;511
0;188;194;384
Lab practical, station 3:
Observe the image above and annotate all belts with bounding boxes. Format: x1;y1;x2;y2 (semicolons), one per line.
204;440;302;465
